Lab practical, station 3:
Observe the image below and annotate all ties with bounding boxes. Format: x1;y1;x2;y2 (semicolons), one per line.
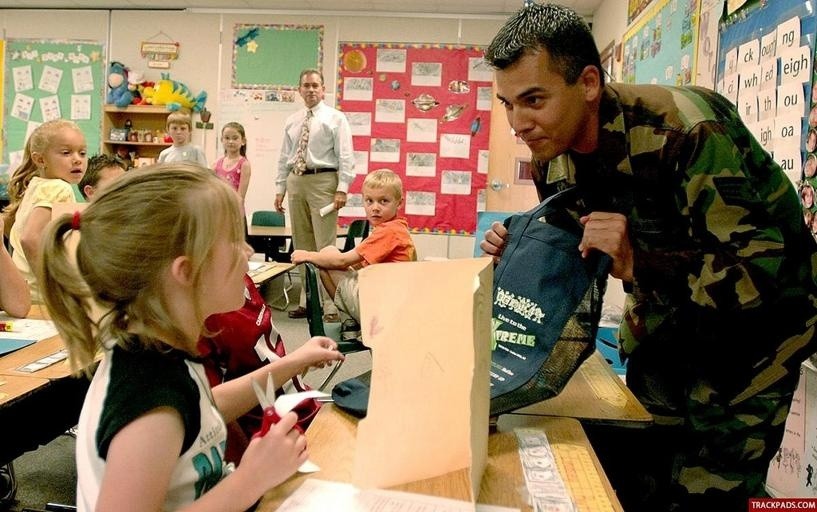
294;110;312;176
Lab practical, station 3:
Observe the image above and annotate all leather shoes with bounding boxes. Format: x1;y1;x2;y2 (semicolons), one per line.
289;306;307;317
324;314;341;322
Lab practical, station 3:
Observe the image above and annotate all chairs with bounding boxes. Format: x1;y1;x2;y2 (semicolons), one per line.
303;262;370;392
337;218;370;253
250;211;286;252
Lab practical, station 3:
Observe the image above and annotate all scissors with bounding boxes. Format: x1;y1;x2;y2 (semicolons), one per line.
250;370;307;455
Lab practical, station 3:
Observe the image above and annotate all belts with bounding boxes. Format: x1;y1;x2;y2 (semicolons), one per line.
303;168;337;174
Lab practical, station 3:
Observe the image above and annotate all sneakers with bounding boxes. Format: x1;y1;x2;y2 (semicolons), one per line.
341;319;360;341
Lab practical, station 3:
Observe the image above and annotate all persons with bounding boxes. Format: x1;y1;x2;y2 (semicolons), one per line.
270;67;359;320
36;160;349;512
474;0;816;512
0;110;421;350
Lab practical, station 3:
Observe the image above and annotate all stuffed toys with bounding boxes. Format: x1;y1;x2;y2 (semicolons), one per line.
102;59;210;118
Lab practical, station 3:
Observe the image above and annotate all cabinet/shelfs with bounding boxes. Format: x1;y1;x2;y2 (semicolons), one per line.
100;104;192;159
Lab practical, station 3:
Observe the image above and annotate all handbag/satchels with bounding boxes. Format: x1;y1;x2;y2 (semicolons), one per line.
332;187;611;415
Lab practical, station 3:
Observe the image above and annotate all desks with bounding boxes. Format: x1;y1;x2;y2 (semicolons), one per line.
503;346;653;432
1;259;299;468
246;225;293;262
253;402;626;512
